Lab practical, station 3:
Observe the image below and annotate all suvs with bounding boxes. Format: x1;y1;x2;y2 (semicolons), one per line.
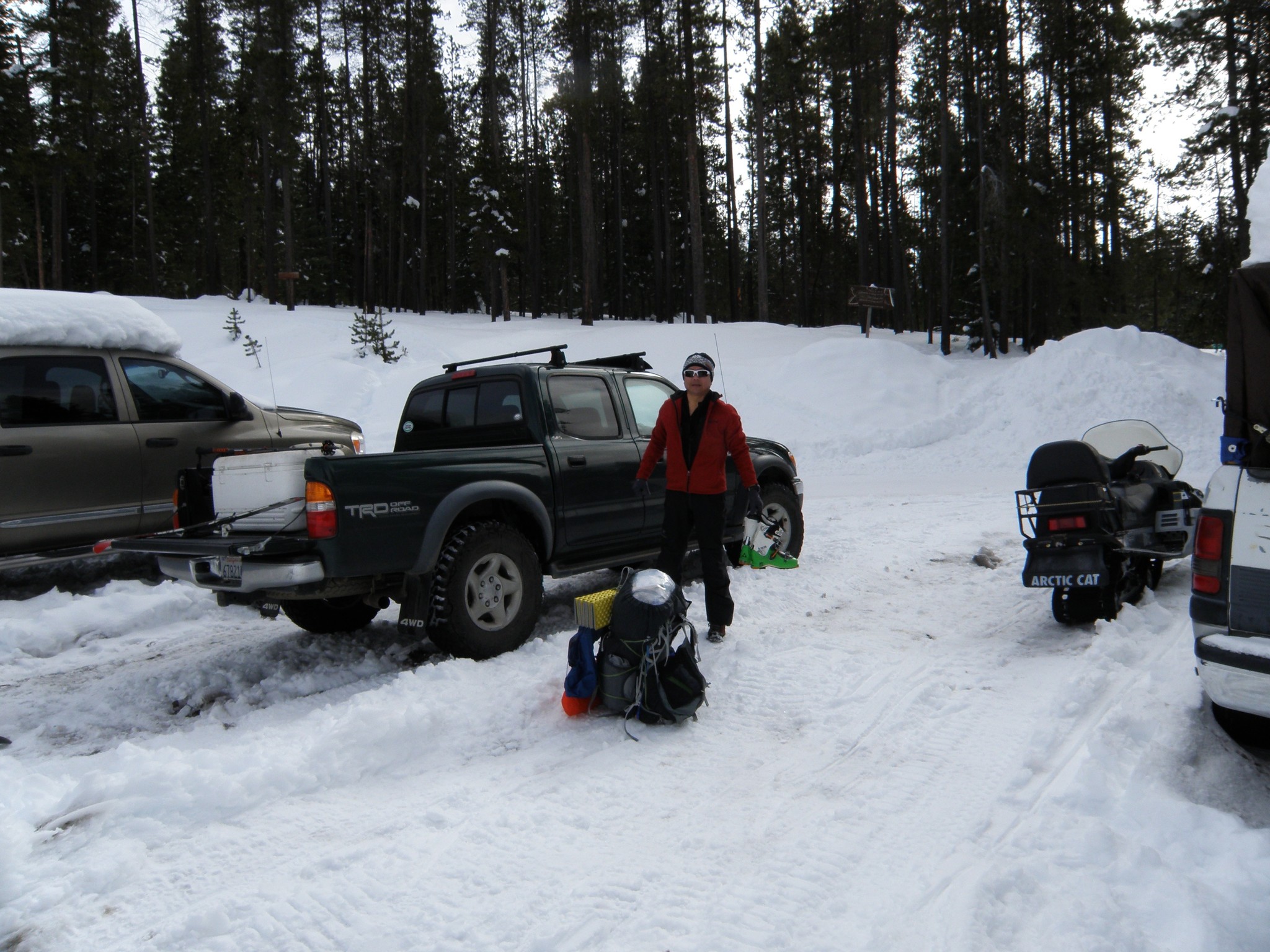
1191;253;1270;748
0;288;364;586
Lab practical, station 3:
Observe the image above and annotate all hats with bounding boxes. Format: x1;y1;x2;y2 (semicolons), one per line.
682;352;715;383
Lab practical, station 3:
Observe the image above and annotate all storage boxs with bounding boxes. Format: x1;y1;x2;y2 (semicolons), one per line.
210;449;346;532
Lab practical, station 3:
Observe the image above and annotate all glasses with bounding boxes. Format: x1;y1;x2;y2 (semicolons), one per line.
683;369;710;378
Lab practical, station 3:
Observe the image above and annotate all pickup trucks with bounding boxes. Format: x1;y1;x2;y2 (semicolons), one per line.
94;344;803;660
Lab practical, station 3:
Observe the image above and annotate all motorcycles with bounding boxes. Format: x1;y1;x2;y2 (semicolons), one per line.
1015;419;1205;626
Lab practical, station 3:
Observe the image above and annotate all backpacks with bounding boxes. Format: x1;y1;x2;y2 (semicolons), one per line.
595;564;693;718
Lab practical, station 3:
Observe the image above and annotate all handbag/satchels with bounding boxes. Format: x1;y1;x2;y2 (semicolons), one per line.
645;636;711;724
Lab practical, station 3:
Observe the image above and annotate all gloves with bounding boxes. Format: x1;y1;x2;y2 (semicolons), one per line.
632;478;651;497
748;488;765;523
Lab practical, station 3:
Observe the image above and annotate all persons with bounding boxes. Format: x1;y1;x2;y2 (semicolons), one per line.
631;352;763;643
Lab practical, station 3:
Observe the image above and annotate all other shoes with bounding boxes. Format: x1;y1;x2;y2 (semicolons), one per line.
707;621;724;642
